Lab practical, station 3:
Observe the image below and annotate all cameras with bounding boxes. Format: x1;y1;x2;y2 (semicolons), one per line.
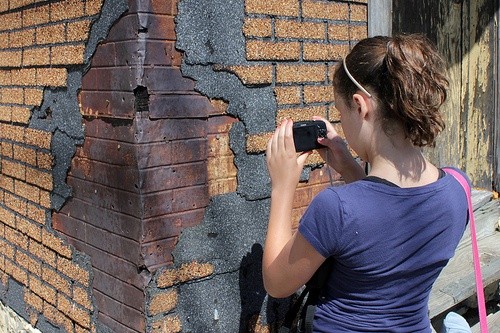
292;122;329;153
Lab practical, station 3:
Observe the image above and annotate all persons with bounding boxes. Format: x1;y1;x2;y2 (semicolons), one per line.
260;32;473;333
